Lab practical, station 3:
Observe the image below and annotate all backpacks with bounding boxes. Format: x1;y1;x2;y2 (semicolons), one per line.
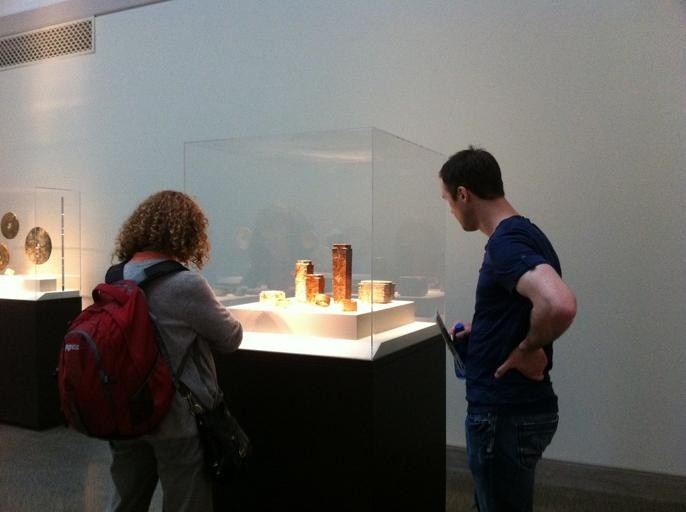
58;259;196;438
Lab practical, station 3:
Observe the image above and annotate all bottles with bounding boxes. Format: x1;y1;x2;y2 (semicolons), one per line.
453;323;468;379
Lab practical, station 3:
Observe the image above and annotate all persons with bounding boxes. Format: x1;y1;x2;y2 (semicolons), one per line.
438;148;577;512
110;190;243;512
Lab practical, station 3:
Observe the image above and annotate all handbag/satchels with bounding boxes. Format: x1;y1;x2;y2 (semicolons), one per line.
197;401;253;485
